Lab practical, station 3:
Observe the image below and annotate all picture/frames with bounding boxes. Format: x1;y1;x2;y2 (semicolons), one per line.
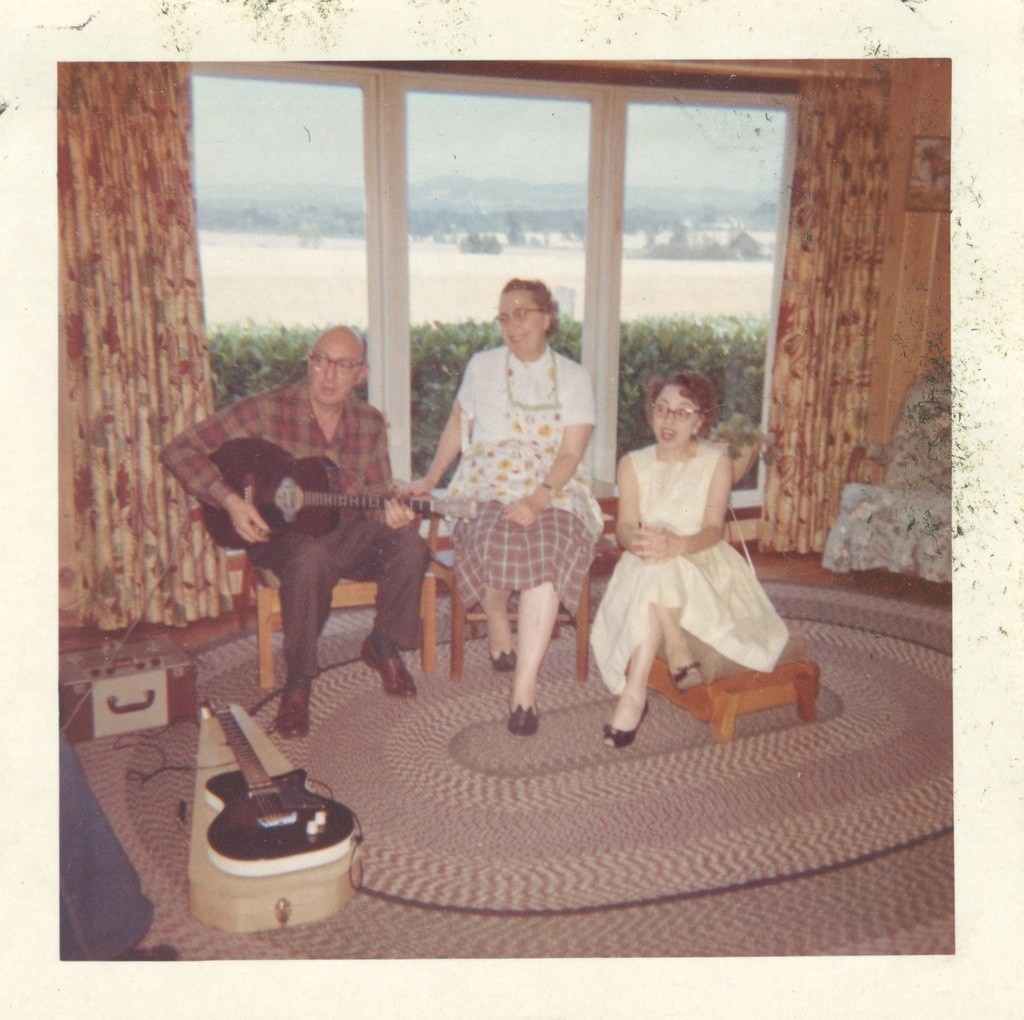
904;133;950;212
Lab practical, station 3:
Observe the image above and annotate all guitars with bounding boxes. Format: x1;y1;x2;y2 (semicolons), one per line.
200;438;480;550
205;692;358;879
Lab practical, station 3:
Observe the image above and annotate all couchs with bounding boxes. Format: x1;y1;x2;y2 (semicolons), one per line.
821;381;952;591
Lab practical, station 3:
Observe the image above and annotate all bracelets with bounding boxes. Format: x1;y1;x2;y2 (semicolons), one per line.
543;483;557;497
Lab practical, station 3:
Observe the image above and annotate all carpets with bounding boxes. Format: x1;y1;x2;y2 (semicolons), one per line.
70;579;956;960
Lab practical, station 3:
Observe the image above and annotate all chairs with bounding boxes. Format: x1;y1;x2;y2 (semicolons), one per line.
426;513;591;677
239;513;435;690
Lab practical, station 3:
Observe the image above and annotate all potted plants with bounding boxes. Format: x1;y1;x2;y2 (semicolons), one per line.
709;413;784;485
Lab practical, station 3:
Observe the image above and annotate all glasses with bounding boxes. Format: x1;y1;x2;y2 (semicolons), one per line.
307;352;364;377
494;306;542;330
650;400;702;420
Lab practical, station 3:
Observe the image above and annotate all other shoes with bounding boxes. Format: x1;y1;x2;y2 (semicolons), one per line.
507;693;541;736
118;940;177;960
486;641;516;670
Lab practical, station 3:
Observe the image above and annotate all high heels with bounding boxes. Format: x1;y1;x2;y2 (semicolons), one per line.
667;660;703;695
600;697;649;748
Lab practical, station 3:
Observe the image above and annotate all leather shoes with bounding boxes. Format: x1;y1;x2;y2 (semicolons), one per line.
361;636;417;699
277;676;313;739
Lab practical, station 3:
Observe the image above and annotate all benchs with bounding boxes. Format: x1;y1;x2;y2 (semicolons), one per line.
621;625;821;742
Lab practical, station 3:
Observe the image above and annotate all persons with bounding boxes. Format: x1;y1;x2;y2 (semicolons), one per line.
159;326;428;739
402;278;604;736
589;370;788;747
